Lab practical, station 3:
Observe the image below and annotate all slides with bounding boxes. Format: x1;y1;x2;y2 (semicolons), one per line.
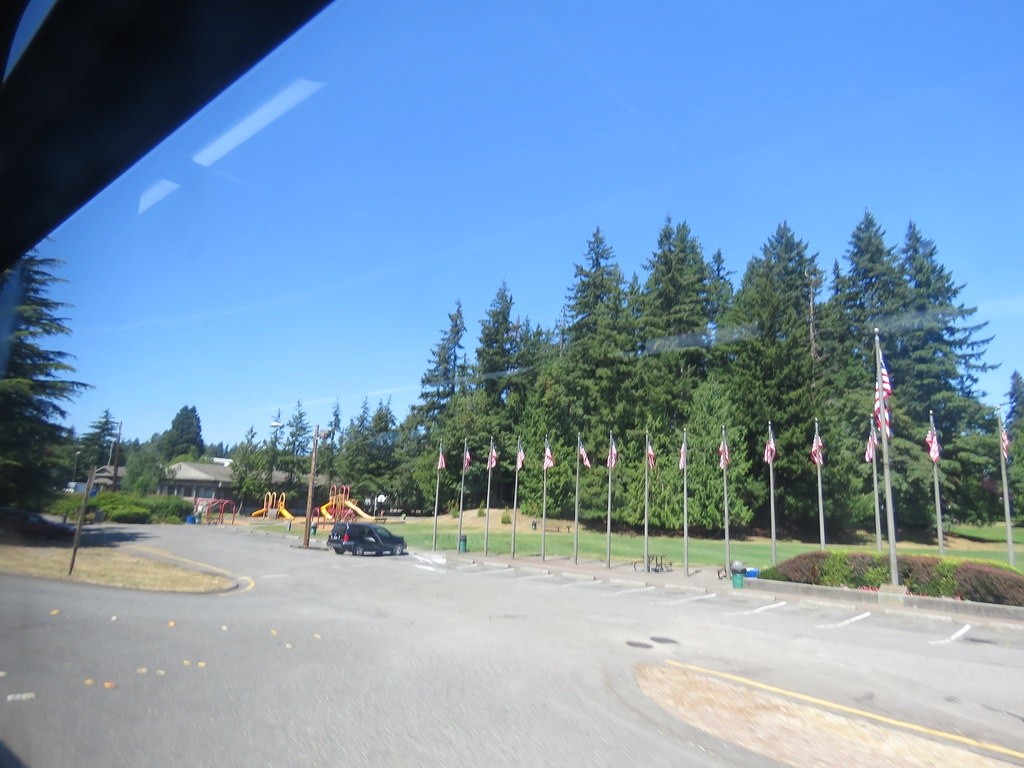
321;502;334;520
251;508;266;516
279;508;294;520
344;500;375;522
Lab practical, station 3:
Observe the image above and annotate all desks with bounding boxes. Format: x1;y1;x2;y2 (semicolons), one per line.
644;554;666;572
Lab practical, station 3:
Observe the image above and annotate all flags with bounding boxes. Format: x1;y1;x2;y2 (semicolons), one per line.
580;437;592;468
517;441;525;470
1001;426;1009;459
864;426;879;463
875;350;894;439
487;442;497;470
810;432;824;466
648;440;655;469
925;422;940;463
763;432;776;465
719;439;730;468
465;445;470;470
438;444;446;470
606;438;617;468
679;443;685;470
543;441;554;471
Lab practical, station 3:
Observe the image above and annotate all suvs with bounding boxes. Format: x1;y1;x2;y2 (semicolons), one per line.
326;522;408;556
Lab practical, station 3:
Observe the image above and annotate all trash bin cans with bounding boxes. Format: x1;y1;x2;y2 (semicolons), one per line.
193;512;202;525
459;535;467;553
310;523;317;535
531;520;537;530
730;566;746;588
